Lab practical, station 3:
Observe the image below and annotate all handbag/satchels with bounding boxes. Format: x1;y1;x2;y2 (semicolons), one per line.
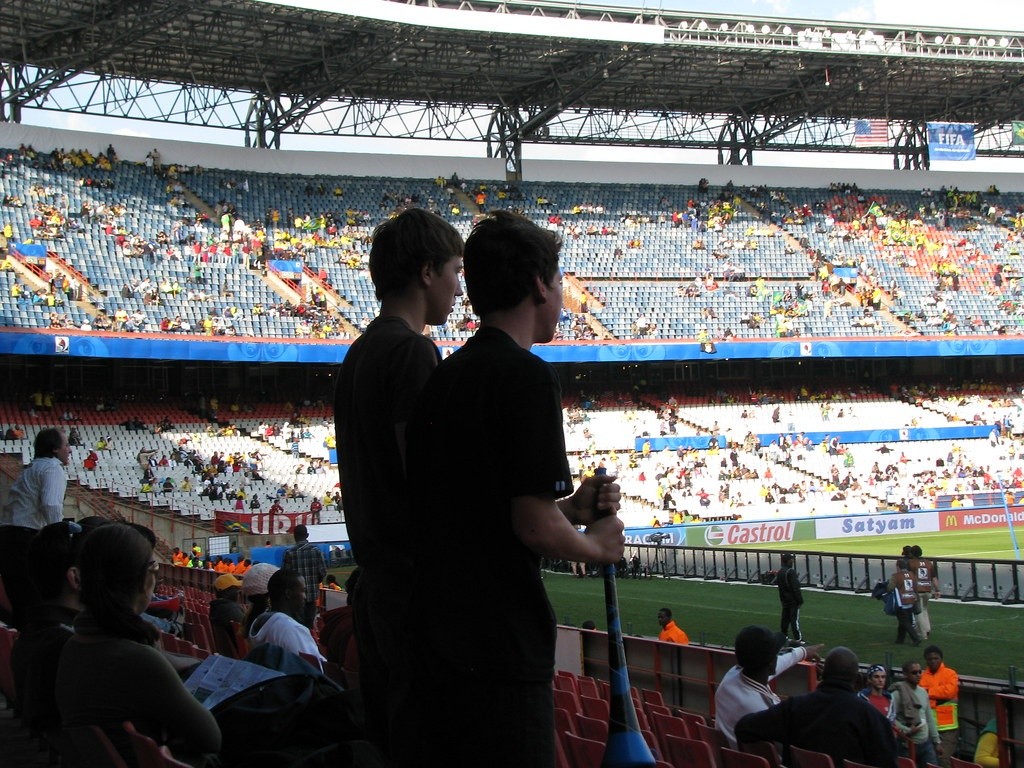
913;602;921;614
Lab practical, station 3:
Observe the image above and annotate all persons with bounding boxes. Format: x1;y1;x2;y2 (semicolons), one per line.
282;525;327;628
405;212;625;768
3;137;1024;344
1;428;72;621
654;607;689;647
206;564;327;669
712;628;958;768
14;521;221;768
776;550;804;649
4;389;1024;525
332;211;466;768
869;545;936;646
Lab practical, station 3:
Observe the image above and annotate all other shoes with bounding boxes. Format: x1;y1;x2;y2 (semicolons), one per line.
894;639;903;643
912;639;921;645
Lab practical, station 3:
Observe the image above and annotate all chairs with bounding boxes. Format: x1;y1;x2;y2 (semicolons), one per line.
0;564;978;768
0;146;1024;527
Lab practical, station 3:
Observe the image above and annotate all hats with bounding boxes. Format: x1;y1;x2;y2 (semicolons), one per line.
214;573;242;591
781;553;796;560
867;664;886;676
243;562;281;595
734;624;788;670
901;546;912;555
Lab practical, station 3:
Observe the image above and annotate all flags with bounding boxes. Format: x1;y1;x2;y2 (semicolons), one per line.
1010;120;1024;145
854;119;887;149
926;122;976;161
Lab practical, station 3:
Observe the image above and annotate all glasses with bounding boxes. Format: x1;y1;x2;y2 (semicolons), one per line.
906;669;922;674
148;560;161;577
